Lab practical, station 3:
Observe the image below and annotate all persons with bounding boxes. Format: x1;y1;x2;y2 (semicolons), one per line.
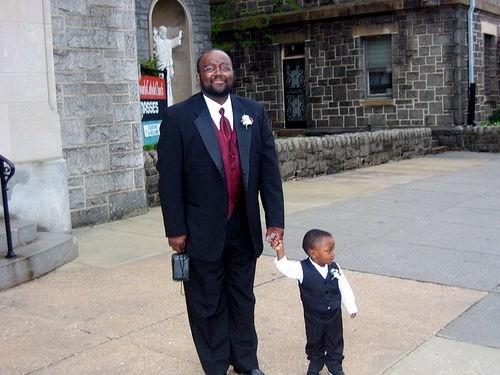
157;48;287;374
268;230;357;375
152;25;183;108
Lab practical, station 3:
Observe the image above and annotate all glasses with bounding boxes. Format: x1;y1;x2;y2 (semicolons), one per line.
201;64;233;74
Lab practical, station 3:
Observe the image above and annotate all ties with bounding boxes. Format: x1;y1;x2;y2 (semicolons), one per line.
218;110;232;145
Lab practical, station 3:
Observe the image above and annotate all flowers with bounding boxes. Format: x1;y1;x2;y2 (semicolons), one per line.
240;113;254;130
331;268;341;280
